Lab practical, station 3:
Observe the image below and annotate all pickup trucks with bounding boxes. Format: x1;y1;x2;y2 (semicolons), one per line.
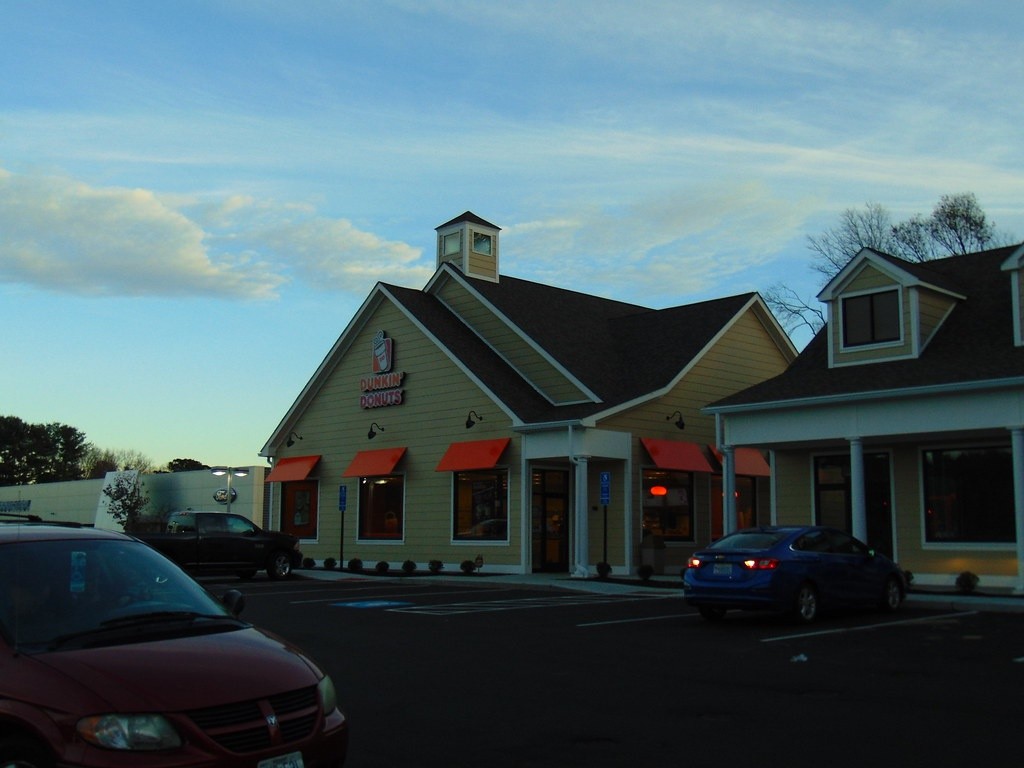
119;510;303;582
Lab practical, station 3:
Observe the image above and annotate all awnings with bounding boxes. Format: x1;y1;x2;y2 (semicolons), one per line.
707;444;771;478
638;436;715;473
435;436;511;472
265;454;322;481
340;447;408;477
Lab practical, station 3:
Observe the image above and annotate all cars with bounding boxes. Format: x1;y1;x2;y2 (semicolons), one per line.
682;525;913;624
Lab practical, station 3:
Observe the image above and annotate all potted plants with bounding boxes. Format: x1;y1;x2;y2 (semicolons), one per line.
402;560;417;571
596;562;613;577
428;560;445;571
460;560;476;574
641;565;653;580
303;558;316;568
376;561;389;573
904;570;914;587
955;571;981;595
324;557;337;569
348;558;363;569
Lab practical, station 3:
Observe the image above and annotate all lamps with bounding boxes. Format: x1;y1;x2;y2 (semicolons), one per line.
286;432;303;448
666;411;685;429
368;422;384;439
375;479;388;484
466;411;483;429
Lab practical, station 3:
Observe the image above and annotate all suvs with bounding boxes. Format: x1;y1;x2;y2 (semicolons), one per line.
0;512;350;768
